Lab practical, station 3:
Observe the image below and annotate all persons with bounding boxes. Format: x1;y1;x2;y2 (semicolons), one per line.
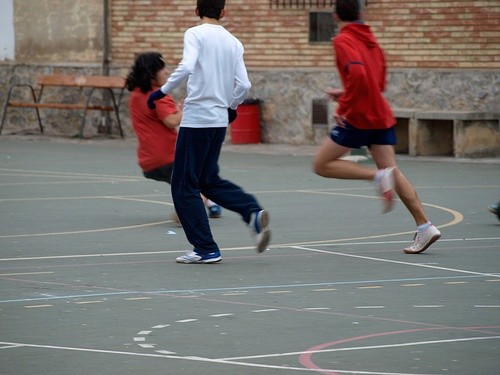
125;51;221;217
313;0;441;254
147;0;271;263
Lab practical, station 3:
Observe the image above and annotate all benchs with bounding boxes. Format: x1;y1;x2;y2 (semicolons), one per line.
393;109;500;158
0;74;127;139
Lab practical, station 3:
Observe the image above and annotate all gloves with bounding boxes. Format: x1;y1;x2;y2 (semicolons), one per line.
147;89;167;109
227;107;237;123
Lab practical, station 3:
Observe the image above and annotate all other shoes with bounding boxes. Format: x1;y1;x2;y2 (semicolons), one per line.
208;205;221;218
488;202;500;213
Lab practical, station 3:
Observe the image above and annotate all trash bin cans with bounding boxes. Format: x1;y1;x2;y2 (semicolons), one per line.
230;98;264;144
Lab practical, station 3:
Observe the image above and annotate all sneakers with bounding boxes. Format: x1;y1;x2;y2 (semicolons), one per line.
375;167;395;215
403;223;441;253
249;210;271;254
176;247;223;263
